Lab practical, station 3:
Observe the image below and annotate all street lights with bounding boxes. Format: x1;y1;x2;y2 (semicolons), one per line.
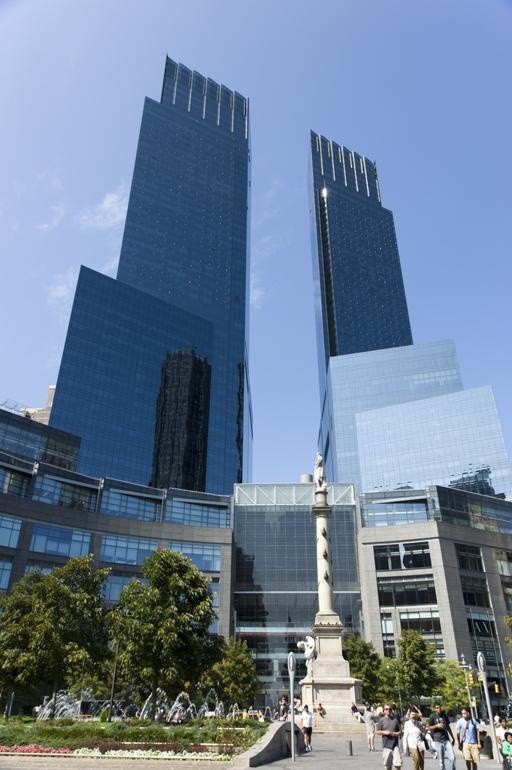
477;651;500;764
287;652;297;763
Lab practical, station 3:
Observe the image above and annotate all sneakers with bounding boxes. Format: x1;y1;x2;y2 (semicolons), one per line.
305;746;312;752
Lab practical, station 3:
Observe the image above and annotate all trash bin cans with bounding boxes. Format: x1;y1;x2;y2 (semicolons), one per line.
235;712;242;721
249;711;258;719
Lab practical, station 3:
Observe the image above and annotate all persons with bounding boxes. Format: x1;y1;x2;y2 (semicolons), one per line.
277;694;325;753
350;701;512;770
314;451;328;492
